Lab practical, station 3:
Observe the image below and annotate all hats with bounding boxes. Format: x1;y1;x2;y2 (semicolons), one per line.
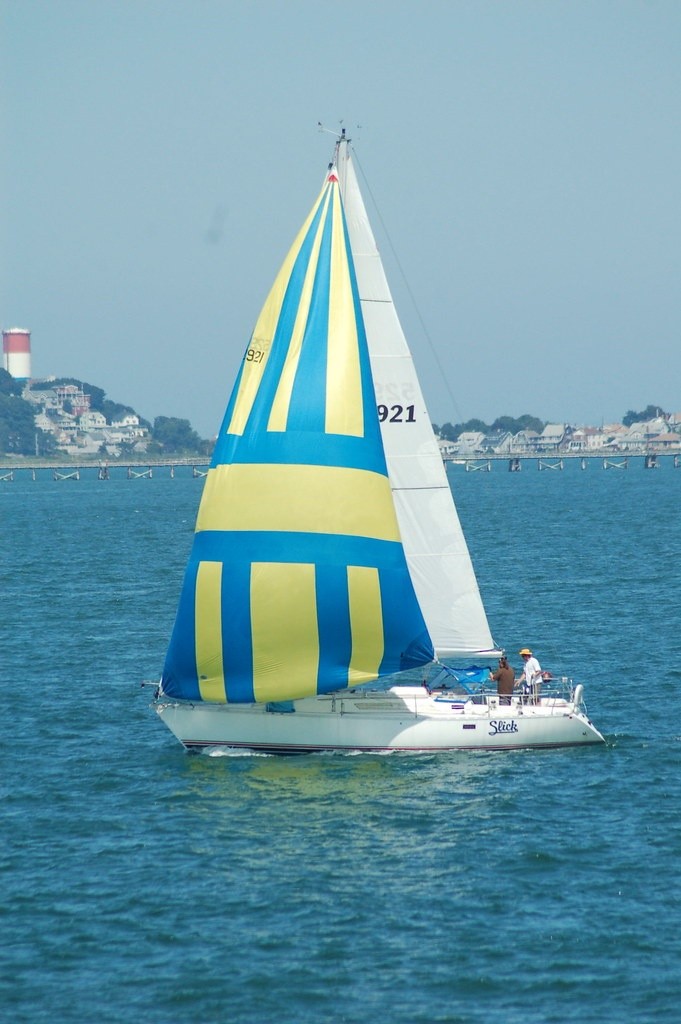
519;648;532;654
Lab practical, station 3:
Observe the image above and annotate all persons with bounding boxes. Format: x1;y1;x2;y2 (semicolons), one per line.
515;648;543;706
489;657;515;706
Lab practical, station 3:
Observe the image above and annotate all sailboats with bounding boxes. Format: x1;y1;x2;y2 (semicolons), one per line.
143;115;610;756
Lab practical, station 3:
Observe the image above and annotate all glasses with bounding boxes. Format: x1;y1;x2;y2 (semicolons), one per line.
522;655;525;657
